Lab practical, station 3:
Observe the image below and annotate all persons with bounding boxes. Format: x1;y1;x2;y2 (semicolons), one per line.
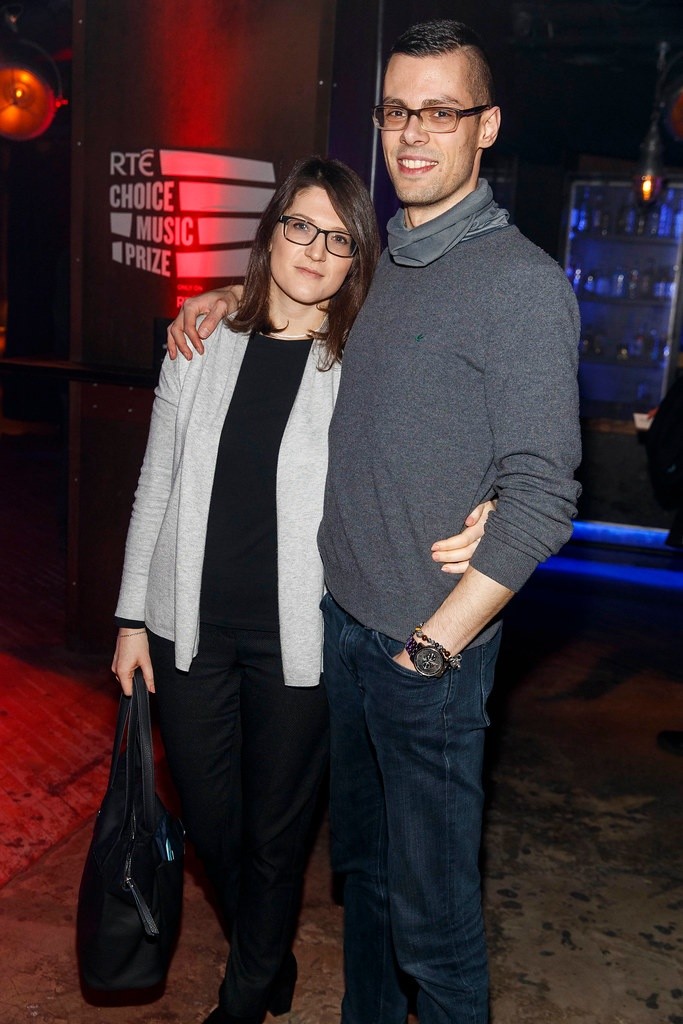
164;20;584;1024
110;153;492;1024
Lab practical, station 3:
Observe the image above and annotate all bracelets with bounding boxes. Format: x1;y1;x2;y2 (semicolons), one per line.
117;631;147;640
415;626;450;658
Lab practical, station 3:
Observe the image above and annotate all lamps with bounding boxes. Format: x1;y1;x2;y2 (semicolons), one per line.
0;36;68;141
632;40;683;207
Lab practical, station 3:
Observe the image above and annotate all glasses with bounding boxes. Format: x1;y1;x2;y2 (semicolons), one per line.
369;104;491;133
278;215;358;258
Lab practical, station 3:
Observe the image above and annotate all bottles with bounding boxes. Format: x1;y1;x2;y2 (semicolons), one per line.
567;186;683;362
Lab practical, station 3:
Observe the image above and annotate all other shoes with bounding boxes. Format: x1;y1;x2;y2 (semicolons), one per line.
205;1011;266;1024
260;976;299;1017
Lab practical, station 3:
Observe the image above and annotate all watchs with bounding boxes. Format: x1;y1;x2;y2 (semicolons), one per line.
404;638;449;678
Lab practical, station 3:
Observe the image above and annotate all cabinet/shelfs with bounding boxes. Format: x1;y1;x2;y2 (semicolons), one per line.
563;182;683;404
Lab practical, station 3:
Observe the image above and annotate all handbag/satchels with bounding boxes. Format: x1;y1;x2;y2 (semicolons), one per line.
77;669;185;1008
